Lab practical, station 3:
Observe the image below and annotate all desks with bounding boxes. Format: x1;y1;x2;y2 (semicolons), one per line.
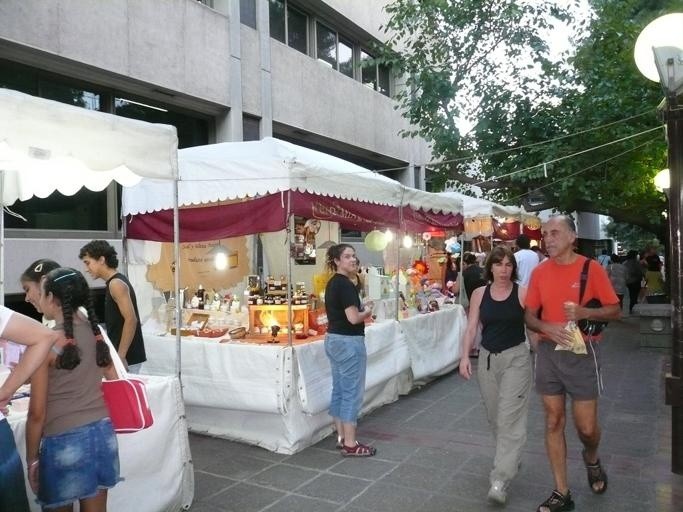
0;372;194;511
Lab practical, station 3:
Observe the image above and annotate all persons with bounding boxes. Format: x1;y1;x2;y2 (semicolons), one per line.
322;244;379;458
22;265;125;512
522;213;623;512
79;237;149;378
445;231;666;359
345;252;369;298
0;301;60;512
456;244;541;511
18;255;93;334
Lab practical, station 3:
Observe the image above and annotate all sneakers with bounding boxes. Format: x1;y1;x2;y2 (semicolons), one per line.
342;441;375;456
488;480;509;505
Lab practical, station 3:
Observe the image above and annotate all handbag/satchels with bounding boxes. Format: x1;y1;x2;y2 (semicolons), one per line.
454;272;470;308
579;257;607;336
97;323;154;433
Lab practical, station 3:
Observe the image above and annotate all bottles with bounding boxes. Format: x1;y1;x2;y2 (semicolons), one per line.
198;285;204;309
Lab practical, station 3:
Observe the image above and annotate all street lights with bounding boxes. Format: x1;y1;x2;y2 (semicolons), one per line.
632;11;682;481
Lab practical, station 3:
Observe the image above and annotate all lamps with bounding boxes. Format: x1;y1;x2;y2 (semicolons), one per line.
364;226;388;252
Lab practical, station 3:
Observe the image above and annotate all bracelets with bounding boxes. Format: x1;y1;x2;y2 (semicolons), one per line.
2;382;15;397
26;458;41;471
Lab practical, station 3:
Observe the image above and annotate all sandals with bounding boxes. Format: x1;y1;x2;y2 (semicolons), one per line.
536;489;574;512
582;449;607;493
